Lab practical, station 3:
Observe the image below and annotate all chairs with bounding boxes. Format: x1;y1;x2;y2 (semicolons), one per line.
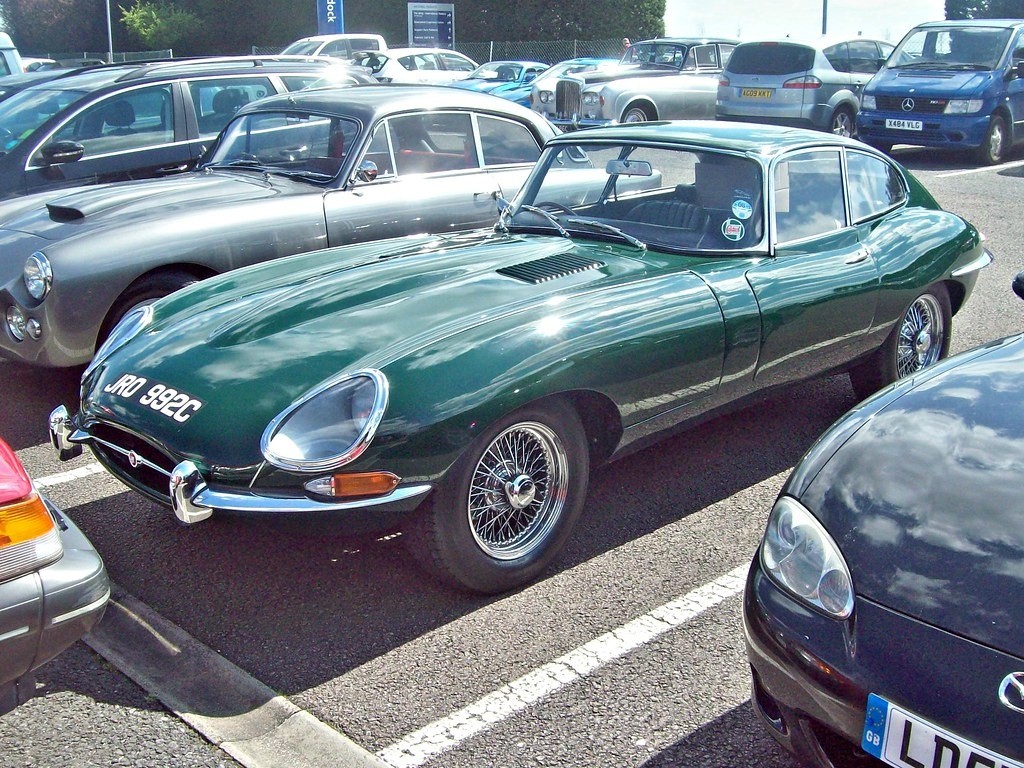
103;101;141;150
153;101;166;136
623;184;716;236
364;153;394;176
37;100;59;129
500;69;516;80
199;89;237;134
693;47;712;64
423;62;435;70
307;155;341;176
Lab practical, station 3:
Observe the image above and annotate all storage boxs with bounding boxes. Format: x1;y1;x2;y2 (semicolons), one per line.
695;161;790;213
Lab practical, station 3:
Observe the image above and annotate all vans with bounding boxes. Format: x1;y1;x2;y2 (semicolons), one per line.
280;33;387;61
855;20;1024;164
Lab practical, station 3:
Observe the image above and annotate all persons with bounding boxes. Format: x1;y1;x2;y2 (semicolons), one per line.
622;37;636;63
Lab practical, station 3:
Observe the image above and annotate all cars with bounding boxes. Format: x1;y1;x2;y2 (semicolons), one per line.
48;118;991;594
741;272;1023;767
349;47;479;86
528;37;742;133
448;61;551;110
0;83;662;370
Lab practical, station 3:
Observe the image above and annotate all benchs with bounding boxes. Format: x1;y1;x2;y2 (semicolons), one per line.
397;149;526;176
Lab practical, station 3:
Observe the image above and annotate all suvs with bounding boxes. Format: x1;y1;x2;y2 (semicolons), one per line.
0;54;380;205
714;33;918;140
